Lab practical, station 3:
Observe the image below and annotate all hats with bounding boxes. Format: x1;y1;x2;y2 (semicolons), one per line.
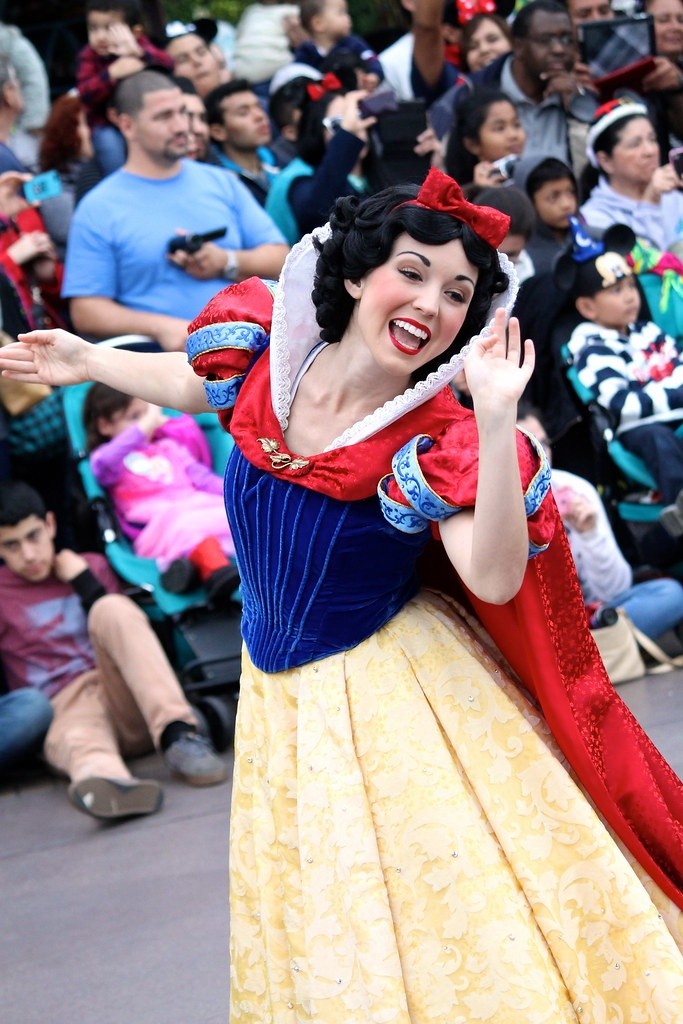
553;223;636;296
586;97;648;170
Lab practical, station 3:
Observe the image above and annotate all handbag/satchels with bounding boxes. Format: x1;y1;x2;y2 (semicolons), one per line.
590;608;683;685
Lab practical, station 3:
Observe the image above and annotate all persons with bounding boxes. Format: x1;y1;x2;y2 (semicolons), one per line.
0;0;683;818
0;167;683;1023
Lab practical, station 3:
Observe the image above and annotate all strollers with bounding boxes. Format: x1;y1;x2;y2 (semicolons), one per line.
556;326;682;587
61;332;245;752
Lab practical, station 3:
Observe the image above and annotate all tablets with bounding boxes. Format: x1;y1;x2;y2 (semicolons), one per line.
594;55;656;105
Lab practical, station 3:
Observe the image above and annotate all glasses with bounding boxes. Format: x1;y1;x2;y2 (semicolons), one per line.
517;30;575;45
322;113;362;136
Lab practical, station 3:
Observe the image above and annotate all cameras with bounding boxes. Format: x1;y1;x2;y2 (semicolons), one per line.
168;227;228;254
484;152;521;188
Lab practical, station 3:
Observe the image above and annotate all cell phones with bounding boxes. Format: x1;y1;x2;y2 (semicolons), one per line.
668;147;683;183
23;171;63;204
359;90;397;120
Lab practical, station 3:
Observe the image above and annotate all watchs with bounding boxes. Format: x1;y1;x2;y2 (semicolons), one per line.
222;248;239;281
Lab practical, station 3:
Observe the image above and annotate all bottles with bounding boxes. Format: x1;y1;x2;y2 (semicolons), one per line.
552;482;599;538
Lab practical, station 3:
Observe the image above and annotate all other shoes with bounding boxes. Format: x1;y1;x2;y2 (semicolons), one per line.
166;732;226;785
660;489;683;538
73;778;163;820
207;566;241;600
160;558;198;595
